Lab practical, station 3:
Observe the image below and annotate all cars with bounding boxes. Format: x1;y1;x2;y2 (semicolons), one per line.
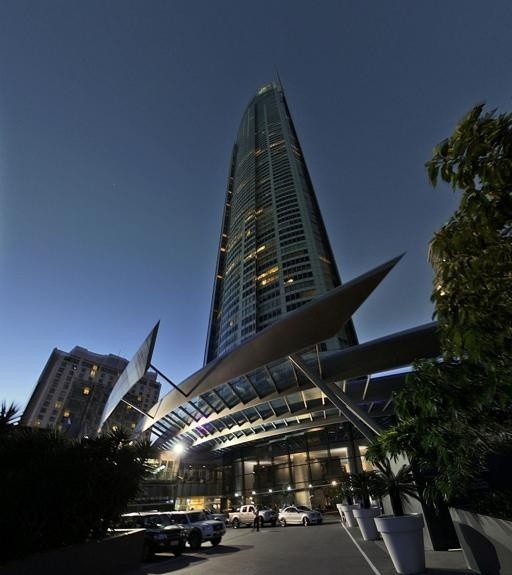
117;504;322;557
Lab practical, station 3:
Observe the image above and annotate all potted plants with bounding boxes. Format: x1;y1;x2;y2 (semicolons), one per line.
338;478;360;527
367;458;428;574
335;490;348;517
342;469;382;541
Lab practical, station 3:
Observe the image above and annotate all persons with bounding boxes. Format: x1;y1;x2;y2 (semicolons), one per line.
250;504;260;532
